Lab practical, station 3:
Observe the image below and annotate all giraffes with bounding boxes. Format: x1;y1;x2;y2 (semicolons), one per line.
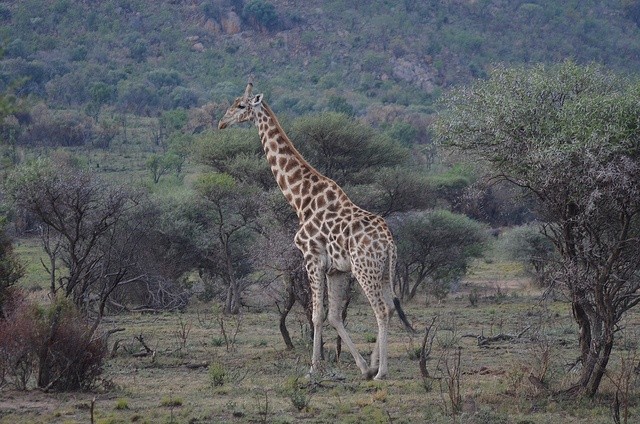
217;82;419;381
